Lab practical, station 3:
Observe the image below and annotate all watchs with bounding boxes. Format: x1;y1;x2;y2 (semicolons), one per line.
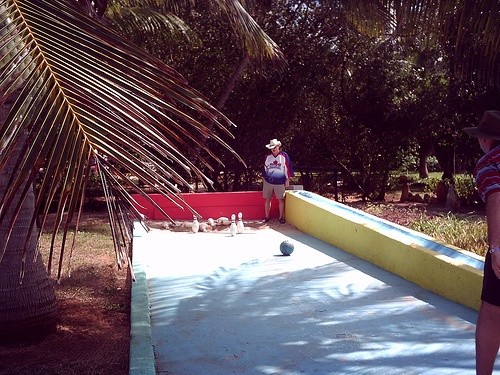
489;247;499;255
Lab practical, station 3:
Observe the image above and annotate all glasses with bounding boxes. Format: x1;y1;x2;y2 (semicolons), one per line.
469;134;483;138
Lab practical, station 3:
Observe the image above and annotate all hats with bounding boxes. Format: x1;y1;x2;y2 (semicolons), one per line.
464;111;500;140
266;138;280;149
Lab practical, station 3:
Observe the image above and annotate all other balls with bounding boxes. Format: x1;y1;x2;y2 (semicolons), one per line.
279;240;294;255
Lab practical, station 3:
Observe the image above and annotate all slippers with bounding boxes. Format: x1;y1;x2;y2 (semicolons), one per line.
262;217;270;223
279;218;285;223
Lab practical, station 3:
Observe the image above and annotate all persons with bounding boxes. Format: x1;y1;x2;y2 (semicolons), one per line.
262;138;295;226
463;110;500;375
